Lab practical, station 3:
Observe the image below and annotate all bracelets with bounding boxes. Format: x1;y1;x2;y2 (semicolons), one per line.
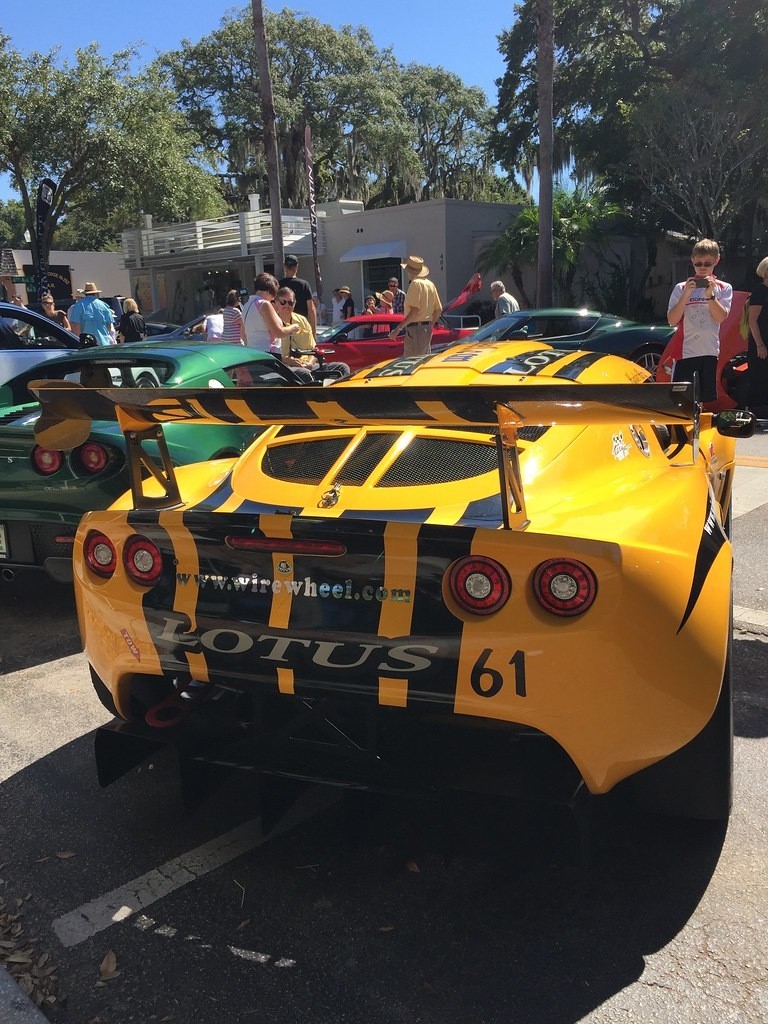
707;294;715;301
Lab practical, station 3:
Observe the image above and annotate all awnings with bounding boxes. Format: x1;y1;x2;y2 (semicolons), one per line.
340;241;407;262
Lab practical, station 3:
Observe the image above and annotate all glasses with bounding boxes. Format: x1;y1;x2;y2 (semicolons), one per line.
389;284;396;287
42;302;54;305
279;299;295;306
693;261;714;267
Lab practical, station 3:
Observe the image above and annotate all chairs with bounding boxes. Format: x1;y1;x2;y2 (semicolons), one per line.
80;368;114;388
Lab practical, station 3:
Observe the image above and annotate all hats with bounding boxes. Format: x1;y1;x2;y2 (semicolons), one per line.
338;286;351;295
70;289;86;297
399;255;431;278
375;290;394;308
81;283;103;294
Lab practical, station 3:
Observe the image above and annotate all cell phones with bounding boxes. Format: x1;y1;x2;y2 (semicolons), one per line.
693;278;709;288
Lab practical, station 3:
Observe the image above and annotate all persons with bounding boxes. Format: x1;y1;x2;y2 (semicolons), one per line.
667;239;733;407
119;299;147;342
748;257;768;433
202;273;350;382
271;255;317;341
313;278;406;336
491;281;519;318
35;283;116;345
0;295;34;339
390;256;443;355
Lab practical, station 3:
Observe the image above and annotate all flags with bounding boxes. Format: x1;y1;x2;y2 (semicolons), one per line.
37;178;57;296
306;125;323;297
443;273;482;313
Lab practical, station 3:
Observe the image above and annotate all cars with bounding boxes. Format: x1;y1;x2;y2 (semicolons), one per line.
0;302;102;386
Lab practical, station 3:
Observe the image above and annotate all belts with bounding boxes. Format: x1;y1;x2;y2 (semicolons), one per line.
407;321;431;327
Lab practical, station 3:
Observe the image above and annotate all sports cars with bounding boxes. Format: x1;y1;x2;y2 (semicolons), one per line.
25;340;760;812
431;308;678;383
0;311;324;588
316;313;477;374
